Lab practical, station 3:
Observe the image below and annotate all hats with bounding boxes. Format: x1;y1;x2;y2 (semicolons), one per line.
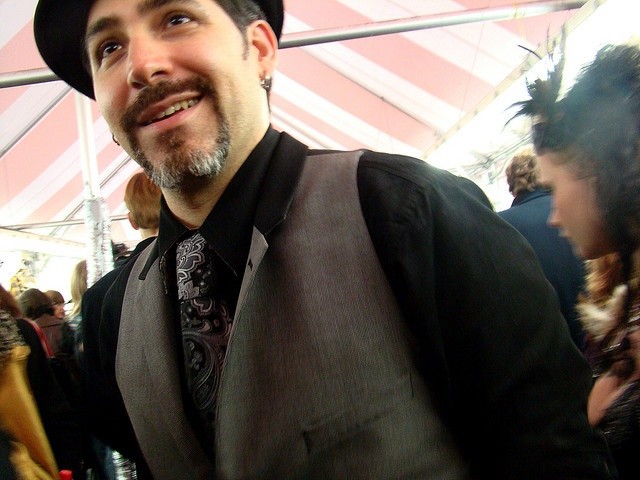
34;1;283;101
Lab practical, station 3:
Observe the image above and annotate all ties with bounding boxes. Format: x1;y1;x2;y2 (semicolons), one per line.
176;233;233;456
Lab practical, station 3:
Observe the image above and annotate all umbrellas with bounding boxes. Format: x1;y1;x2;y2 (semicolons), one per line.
0;0;594;277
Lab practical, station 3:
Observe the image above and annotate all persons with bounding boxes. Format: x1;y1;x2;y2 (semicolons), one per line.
0;258;88;480
533;38;640;479
494;152;589;353
79;169;160;480
35;0;605;478
